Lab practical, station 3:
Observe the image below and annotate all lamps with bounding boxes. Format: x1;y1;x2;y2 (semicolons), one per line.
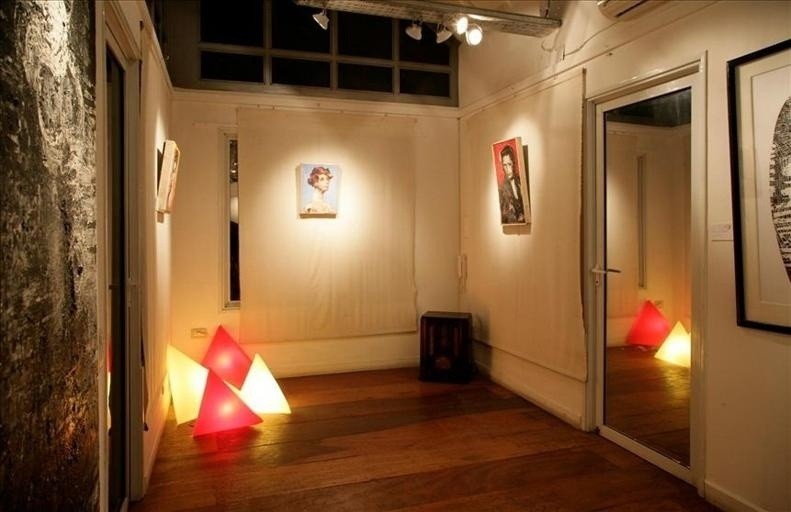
625;299;691;369
312;1;329;31
164;325;291;436
405;13;483;46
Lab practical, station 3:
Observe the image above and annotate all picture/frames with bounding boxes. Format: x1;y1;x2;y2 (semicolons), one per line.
157;139;181;214
725;38;791;335
223;134;241;307
491;137;532;227
300;165;337;217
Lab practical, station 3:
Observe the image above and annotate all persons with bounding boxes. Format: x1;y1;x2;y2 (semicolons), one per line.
300;166;334;215
496;144;526;224
